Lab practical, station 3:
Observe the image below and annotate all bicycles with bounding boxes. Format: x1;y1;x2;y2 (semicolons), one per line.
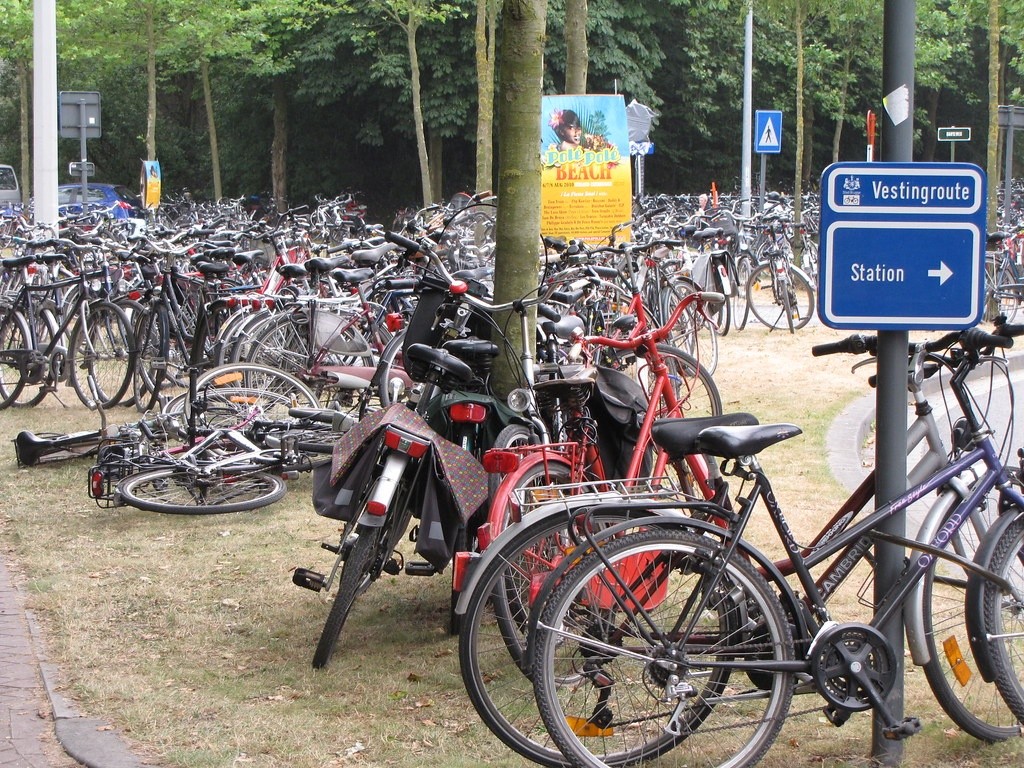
0;187;1024;672
452;281;1024;768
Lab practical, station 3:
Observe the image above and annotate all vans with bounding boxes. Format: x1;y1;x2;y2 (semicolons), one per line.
0;164;22;208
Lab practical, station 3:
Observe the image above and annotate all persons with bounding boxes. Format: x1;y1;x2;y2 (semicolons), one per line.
552;109;601;153
148;165;159;179
695;194;712;212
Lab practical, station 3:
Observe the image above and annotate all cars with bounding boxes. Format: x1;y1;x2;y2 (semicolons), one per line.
29;184;144;219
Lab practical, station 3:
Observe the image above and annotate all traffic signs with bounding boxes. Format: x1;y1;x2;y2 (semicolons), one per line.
818;161;988;329
